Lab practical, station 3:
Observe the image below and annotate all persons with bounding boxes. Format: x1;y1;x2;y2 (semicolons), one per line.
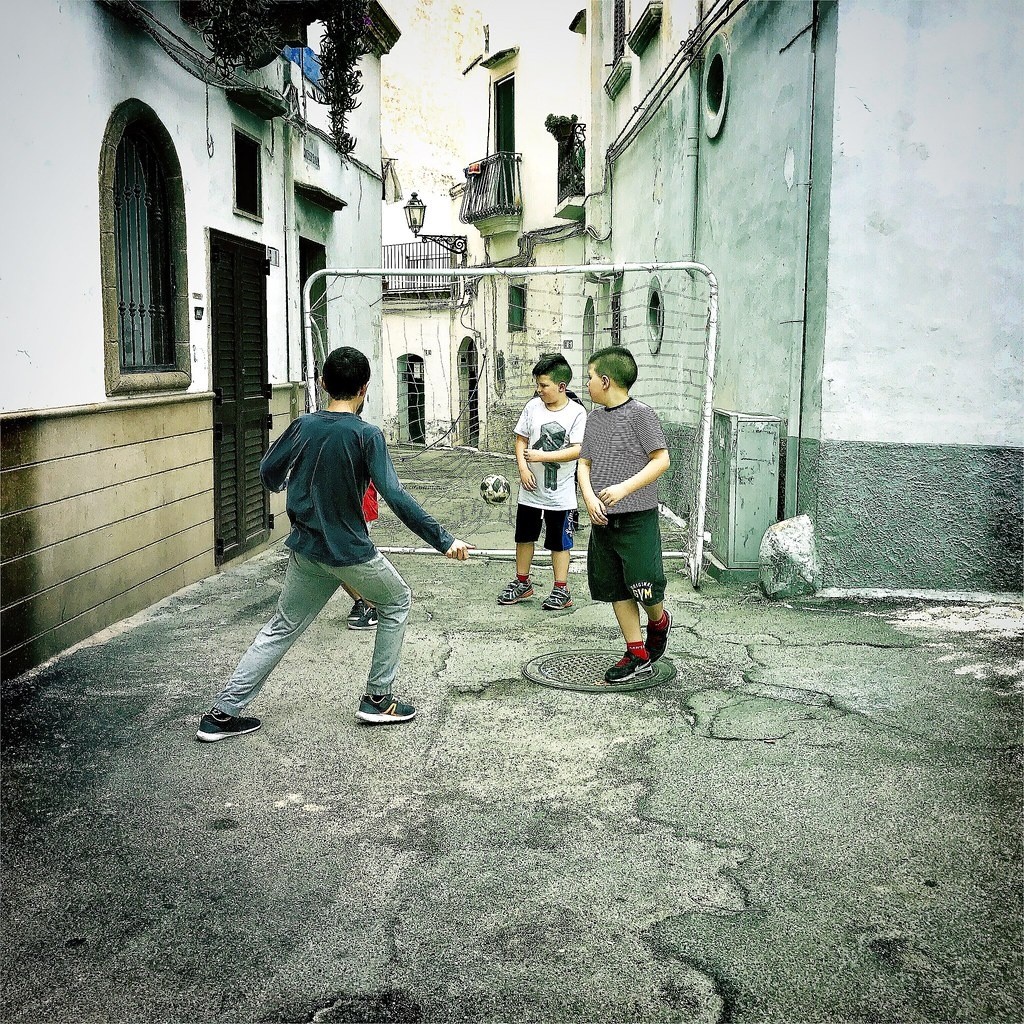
497;353;588;610
196;346;475;742
338;391;382;630
578;347;675;681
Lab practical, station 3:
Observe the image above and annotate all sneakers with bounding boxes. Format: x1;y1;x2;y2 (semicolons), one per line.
542;584;572;611
606;610;673;682
497;580;534;604
354;694;417;723
197;716;261;741
349;599;378;631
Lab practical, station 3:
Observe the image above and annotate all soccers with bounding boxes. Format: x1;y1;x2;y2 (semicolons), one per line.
480;473;510;505
